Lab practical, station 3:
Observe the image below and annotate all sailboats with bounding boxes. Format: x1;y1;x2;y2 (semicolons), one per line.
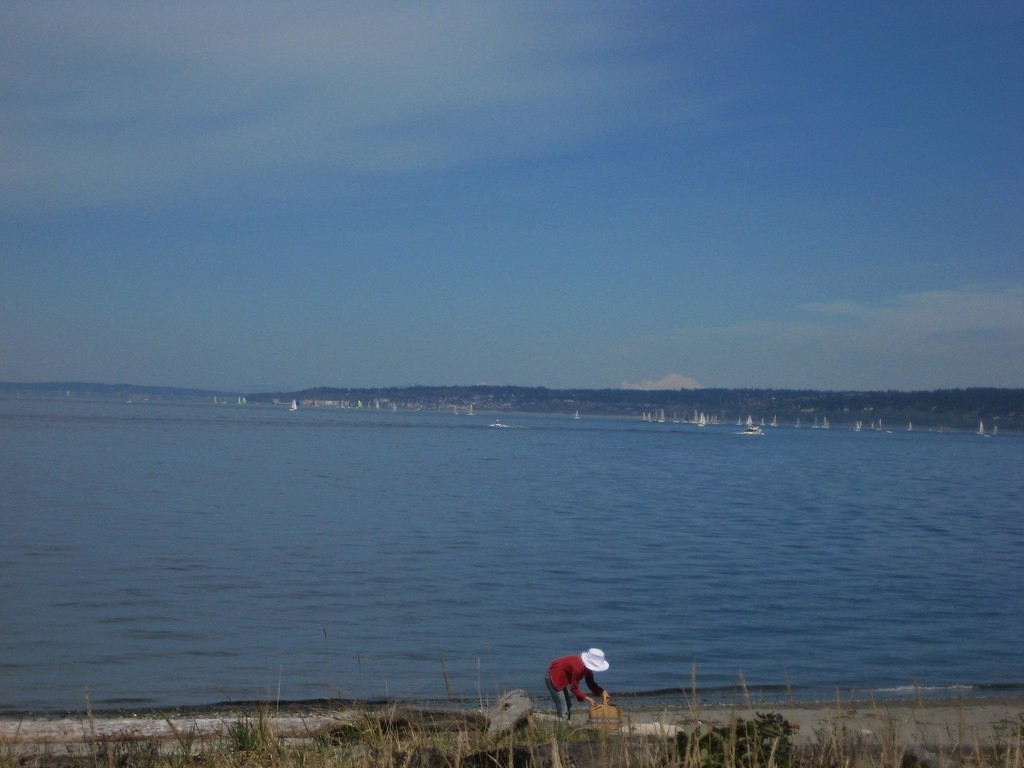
853;420;916;435
237;396;247;406
574;410;580;421
340;399;397;413
976;421;998;437
641;408;666;424
672;408;722;426
737;414;779;426
795;415;831;430
289;399;298;412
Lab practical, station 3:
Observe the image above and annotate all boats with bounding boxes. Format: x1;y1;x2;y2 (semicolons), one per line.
742;424;764;436
495;419;501;428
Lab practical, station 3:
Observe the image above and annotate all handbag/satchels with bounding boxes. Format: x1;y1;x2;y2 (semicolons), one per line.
589;695;621;730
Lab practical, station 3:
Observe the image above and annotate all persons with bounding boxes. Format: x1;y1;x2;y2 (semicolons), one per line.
544;648;611;719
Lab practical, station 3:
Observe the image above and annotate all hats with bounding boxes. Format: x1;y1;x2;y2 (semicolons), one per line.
581;648;610;672
